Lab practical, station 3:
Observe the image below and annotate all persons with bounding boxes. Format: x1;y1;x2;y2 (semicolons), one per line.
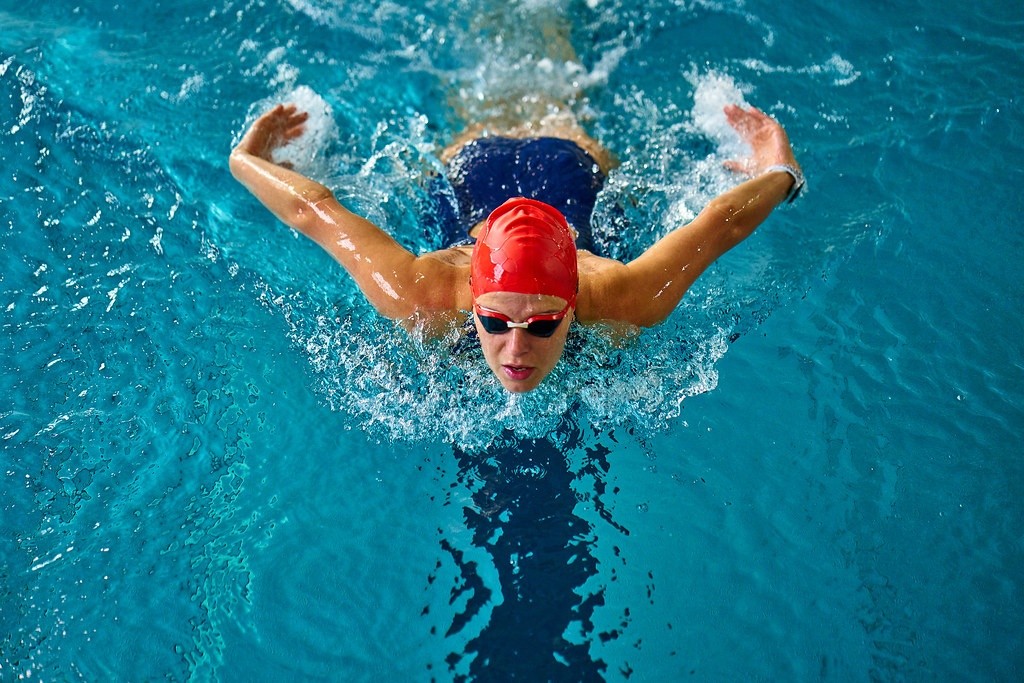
229;12;799;395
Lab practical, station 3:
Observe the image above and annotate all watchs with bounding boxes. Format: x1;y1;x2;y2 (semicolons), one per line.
764;162;806;204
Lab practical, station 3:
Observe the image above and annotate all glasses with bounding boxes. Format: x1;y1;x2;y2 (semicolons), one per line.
470;285;575;338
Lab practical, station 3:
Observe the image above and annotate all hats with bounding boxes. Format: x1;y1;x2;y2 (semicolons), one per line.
469;198;578;311
